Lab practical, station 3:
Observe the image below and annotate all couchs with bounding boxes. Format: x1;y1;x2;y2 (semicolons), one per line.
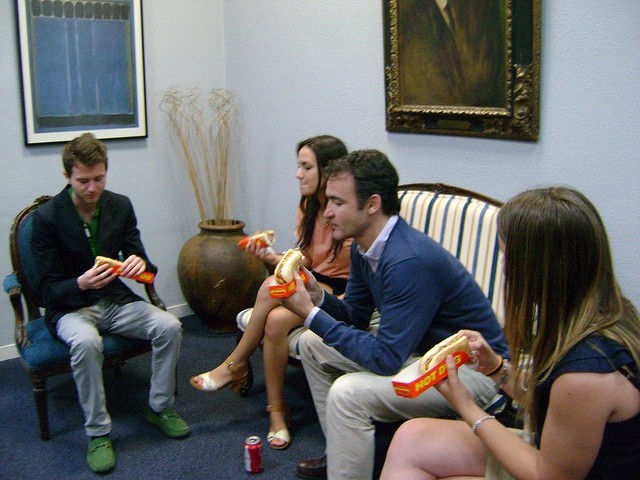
234;183;524;428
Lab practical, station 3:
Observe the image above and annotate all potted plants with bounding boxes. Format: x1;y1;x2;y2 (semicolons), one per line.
158;87;266;333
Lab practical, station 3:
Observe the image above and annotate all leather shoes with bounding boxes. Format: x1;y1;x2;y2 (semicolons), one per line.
297;455;327;476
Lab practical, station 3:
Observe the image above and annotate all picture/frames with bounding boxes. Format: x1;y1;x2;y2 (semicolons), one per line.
382;0;542;142
17;0;148;146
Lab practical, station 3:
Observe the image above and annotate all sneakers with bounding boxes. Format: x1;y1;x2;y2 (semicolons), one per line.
86;438;116;473
146;409;191;439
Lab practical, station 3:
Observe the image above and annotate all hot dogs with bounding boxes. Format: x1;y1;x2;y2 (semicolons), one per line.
419;333;470;375
274;248;302;285
93;256;123;272
250;231;275;246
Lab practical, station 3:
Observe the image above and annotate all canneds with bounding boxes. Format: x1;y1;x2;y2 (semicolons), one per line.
244;436;263;473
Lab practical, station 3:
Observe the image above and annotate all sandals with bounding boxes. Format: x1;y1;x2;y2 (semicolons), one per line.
267;405;290;449
191;358;249;392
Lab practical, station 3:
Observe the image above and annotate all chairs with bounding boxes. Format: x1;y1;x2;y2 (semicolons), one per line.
3;195;177;442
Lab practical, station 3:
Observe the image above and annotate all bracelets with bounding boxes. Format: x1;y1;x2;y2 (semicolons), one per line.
484;356;506;377
471;415;495;430
492;359;509;391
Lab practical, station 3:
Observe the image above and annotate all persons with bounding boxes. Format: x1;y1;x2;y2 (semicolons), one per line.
372;189;640;480
282;150;510;480
189;135;353;450
32;134;190;471
